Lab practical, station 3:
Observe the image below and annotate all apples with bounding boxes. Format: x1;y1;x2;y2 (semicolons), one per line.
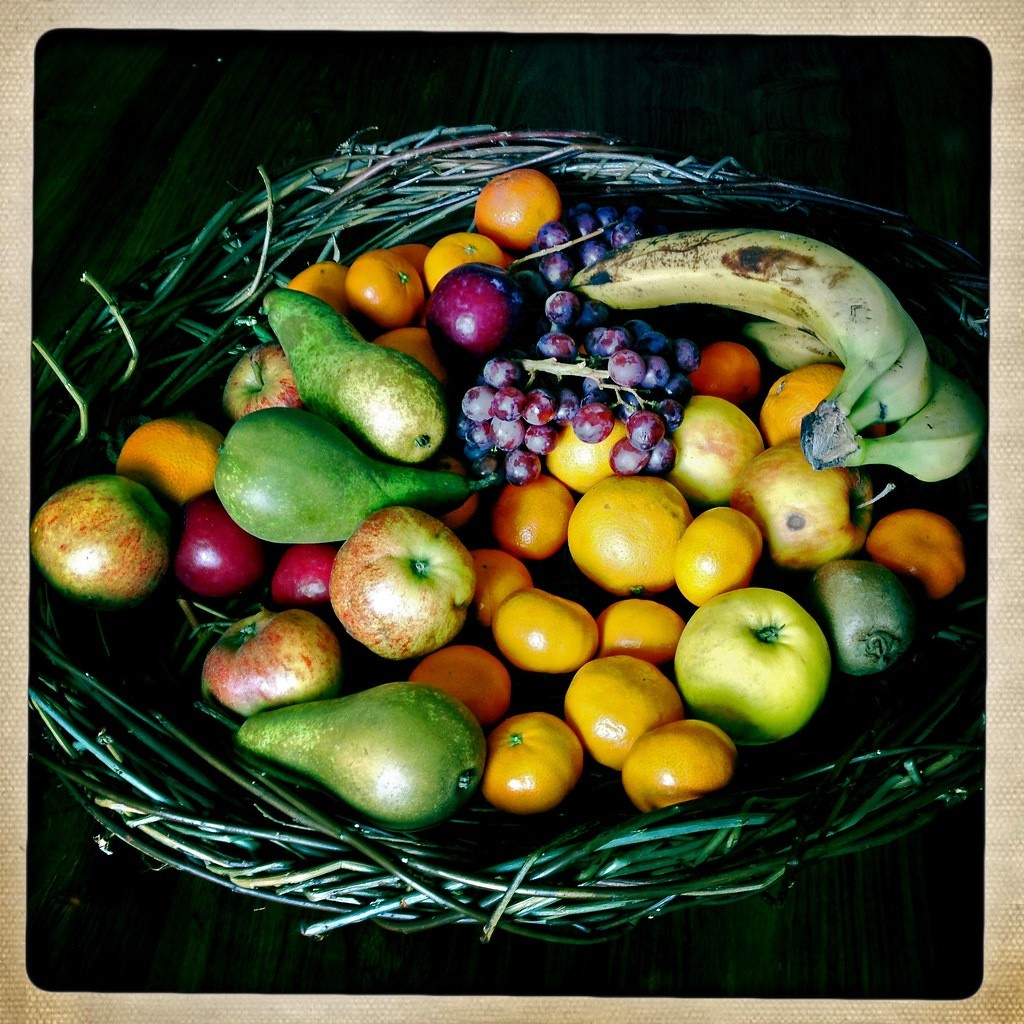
328;507;476;659
29;472;176;604
201;609;343;717
173;489;266;598
269;544;335;609
222;342;302;421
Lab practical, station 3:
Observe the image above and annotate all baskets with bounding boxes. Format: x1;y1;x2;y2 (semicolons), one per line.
30;121;986;952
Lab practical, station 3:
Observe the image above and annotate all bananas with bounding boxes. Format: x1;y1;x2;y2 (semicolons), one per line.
569;227;984;480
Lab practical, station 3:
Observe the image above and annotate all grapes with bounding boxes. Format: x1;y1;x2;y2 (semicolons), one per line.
454;198;702;487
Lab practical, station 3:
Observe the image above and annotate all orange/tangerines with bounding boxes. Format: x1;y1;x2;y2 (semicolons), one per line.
115;413;223;510
284;168;563;391
407;341;966;817
